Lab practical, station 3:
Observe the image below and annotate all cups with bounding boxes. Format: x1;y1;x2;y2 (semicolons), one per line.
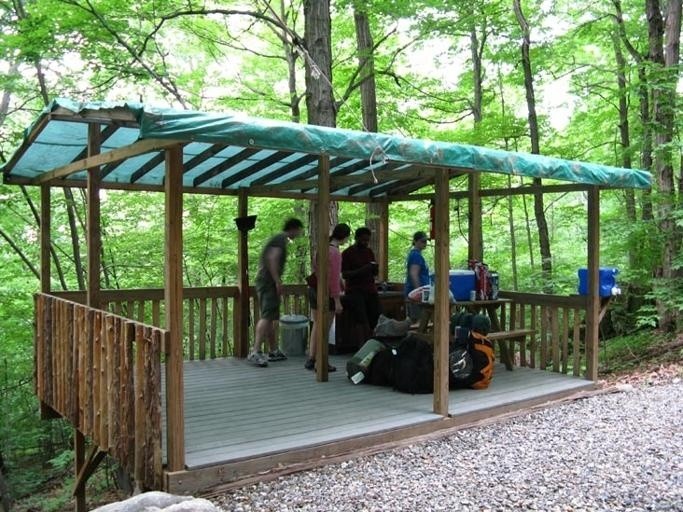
470;291;477;302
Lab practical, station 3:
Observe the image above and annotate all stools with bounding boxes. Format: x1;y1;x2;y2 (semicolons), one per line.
279;315;312;357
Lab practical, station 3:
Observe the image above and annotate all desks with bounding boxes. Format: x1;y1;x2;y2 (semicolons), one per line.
328;291;407;355
396;298;516;371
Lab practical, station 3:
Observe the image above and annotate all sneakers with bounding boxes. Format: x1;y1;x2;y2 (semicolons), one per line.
305;359;336;372
268;350;287;361
248;351;268;366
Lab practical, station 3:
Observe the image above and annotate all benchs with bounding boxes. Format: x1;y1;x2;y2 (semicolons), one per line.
408;322;539;341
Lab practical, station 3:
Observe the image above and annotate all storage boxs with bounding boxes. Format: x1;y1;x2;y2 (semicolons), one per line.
448;269;476;300
578;267;621;299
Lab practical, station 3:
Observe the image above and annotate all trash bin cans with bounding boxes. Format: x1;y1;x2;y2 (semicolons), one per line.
280;314;308;357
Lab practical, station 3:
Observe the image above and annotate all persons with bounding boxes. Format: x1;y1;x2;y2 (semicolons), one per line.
244;218;304;368
340;226;385;355
402;230;430;322
303;223;352;373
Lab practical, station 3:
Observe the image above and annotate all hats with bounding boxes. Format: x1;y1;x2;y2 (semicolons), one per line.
414;231;431;241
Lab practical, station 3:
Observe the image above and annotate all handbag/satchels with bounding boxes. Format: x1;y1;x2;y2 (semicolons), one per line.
305;271;317;289
346;313;495;394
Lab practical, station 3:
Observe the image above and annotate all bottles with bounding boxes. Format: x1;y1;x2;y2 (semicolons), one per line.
422;287;430;303
